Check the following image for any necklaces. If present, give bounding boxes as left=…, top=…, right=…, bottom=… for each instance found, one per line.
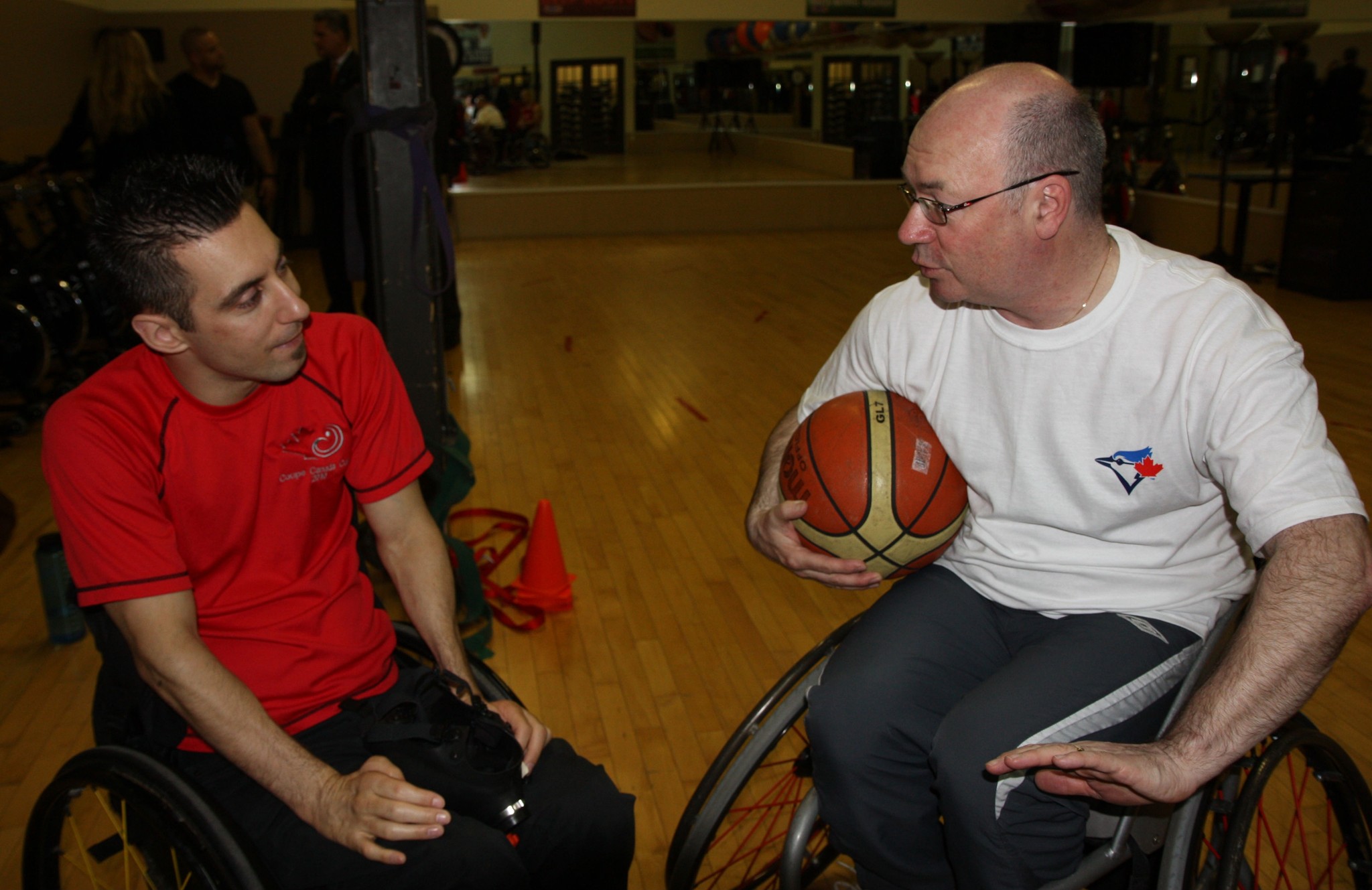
left=1058, top=233, right=1112, bottom=327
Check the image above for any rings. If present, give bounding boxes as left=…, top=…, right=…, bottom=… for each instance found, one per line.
left=1074, top=744, right=1084, bottom=750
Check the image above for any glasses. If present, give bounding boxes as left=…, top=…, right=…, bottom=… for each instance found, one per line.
left=897, top=169, right=1081, bottom=226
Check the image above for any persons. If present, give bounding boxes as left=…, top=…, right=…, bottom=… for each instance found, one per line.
left=40, top=161, right=636, bottom=889
left=741, top=63, right=1372, bottom=890
left=35, top=9, right=1318, bottom=364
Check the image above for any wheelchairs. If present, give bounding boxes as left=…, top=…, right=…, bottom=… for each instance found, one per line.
left=20, top=473, right=553, bottom=890
left=0, top=168, right=135, bottom=442
left=662, top=550, right=1372, bottom=890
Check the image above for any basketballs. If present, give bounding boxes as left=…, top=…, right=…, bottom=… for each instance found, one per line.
left=779, top=390, right=967, bottom=580
